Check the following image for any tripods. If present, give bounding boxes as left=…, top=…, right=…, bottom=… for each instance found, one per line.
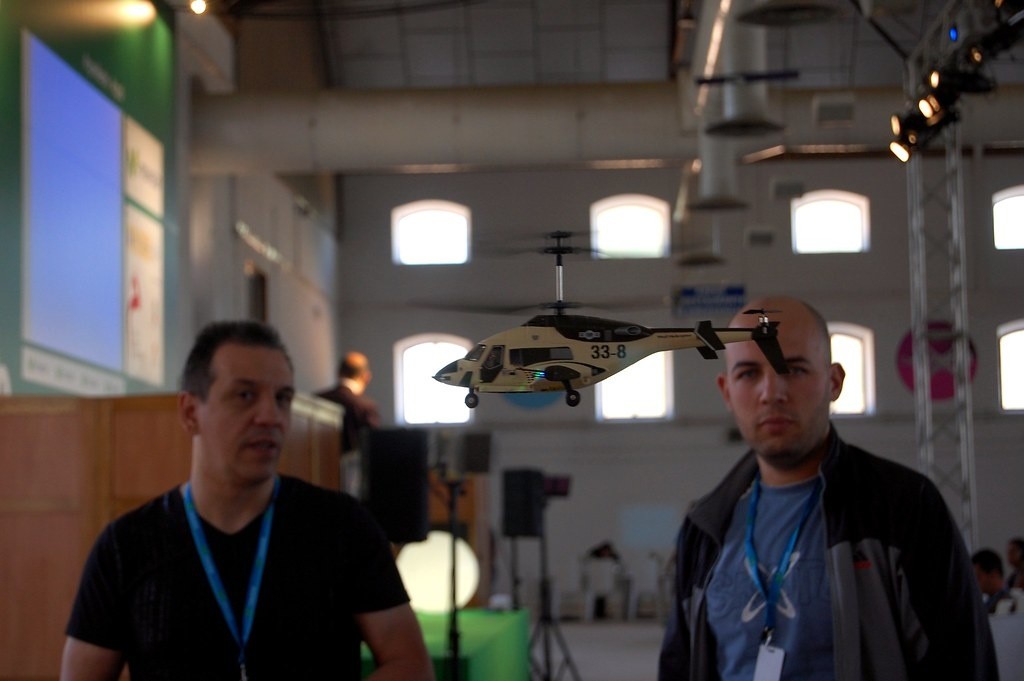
left=528, top=518, right=580, bottom=681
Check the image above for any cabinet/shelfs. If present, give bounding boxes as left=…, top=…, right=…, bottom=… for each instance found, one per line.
left=0, top=386, right=344, bottom=681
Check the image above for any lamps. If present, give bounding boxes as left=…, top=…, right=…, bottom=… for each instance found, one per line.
left=890, top=22, right=1017, bottom=162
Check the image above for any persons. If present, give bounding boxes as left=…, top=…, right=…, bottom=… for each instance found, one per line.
left=972, top=549, right=1015, bottom=612
left=1007, top=539, right=1024, bottom=589
left=658, top=295, right=1002, bottom=681
left=318, top=351, right=381, bottom=449
left=58, top=323, right=435, bottom=681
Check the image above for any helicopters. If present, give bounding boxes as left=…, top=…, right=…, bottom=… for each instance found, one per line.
left=430, top=225, right=794, bottom=407
